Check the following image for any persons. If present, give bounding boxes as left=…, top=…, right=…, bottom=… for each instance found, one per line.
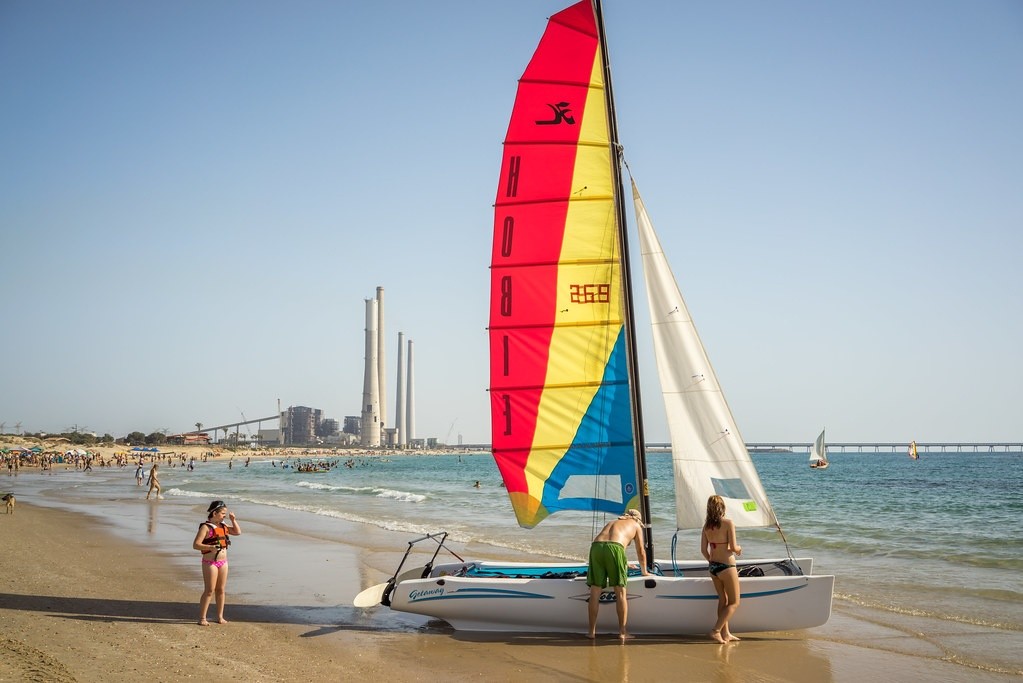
left=74, top=450, right=79, bottom=458
left=245, top=457, right=250, bottom=467
left=229, top=458, right=233, bottom=469
left=459, top=456, right=461, bottom=462
left=146, top=464, right=160, bottom=499
left=473, top=481, right=480, bottom=487
left=251, top=448, right=389, bottom=472
left=501, top=483, right=505, bottom=487
left=193, top=500, right=241, bottom=626
left=135, top=465, right=145, bottom=485
left=585, top=509, right=652, bottom=639
left=817, top=460, right=826, bottom=466
left=0, top=451, right=220, bottom=473
left=701, top=495, right=742, bottom=643
left=2, top=493, right=15, bottom=514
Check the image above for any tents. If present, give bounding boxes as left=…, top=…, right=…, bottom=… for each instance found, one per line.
left=2, top=445, right=42, bottom=453
left=66, top=450, right=75, bottom=455
left=78, top=449, right=87, bottom=455
left=132, top=446, right=159, bottom=451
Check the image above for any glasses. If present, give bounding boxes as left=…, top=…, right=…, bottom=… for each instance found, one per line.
left=211, top=501, right=224, bottom=513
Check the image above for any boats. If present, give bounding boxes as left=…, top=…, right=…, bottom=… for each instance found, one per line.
left=293, top=470, right=328, bottom=473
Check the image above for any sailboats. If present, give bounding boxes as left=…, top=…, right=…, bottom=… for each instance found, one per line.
left=808, top=426, right=829, bottom=469
left=354, top=0, right=832, bottom=633
left=909, top=441, right=919, bottom=460
